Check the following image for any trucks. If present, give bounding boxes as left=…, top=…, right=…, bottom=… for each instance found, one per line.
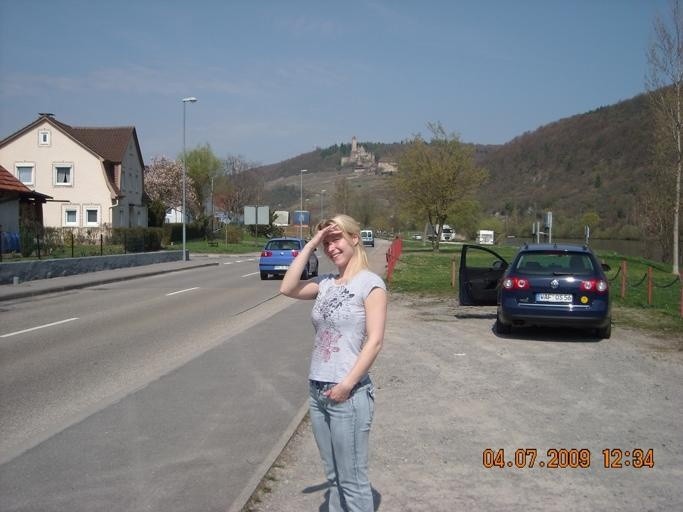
left=426, top=223, right=453, bottom=241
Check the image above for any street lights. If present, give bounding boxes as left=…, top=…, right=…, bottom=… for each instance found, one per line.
left=182, top=96, right=198, bottom=262
left=301, top=169, right=306, bottom=237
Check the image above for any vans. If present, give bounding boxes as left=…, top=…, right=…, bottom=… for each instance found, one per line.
left=360, top=229, right=375, bottom=247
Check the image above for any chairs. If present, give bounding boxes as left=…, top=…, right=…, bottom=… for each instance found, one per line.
left=270, top=244, right=279, bottom=249
left=526, top=255, right=584, bottom=272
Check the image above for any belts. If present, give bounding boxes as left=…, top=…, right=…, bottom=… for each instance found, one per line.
left=308, top=380, right=361, bottom=390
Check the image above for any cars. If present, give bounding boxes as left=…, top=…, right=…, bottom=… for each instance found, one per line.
left=258, top=237, right=319, bottom=280
left=458, top=243, right=611, bottom=340
left=412, top=234, right=422, bottom=240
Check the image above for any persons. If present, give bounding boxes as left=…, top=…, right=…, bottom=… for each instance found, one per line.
left=280, top=213, right=387, bottom=512
